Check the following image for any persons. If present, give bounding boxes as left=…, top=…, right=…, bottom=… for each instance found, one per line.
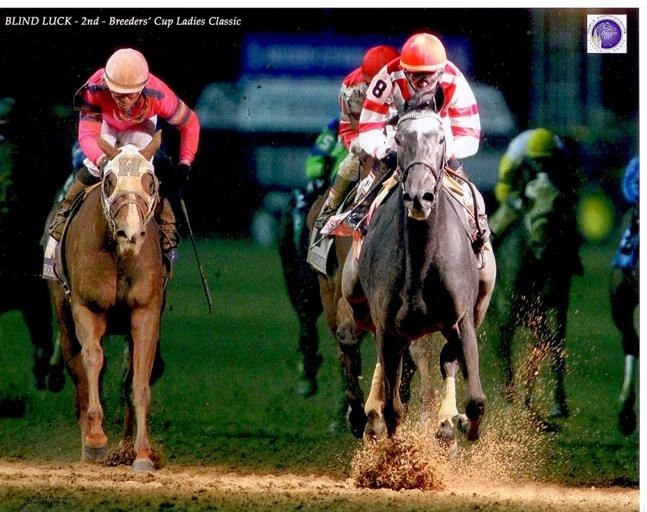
left=611, top=151, right=639, bottom=274
left=348, top=31, right=484, bottom=246
left=41, top=47, right=202, bottom=260
left=307, top=44, right=401, bottom=232
left=485, top=126, right=585, bottom=275
left=296, top=115, right=348, bottom=214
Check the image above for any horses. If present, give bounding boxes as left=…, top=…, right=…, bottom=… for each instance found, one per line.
left=307, top=90, right=433, bottom=438
left=610, top=268, right=640, bottom=436
left=271, top=178, right=363, bottom=399
left=485, top=153, right=577, bottom=433
left=357, top=81, right=497, bottom=462
left=47, top=129, right=164, bottom=473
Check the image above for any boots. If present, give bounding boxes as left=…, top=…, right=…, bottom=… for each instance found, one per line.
left=315, top=174, right=357, bottom=230
left=350, top=159, right=388, bottom=224
left=48, top=175, right=87, bottom=242
left=451, top=162, right=490, bottom=241
left=154, top=181, right=178, bottom=250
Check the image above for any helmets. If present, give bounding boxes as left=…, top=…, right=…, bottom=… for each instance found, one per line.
left=527, top=129, right=553, bottom=157
left=399, top=32, right=447, bottom=73
left=103, top=48, right=150, bottom=94
left=362, top=46, right=401, bottom=77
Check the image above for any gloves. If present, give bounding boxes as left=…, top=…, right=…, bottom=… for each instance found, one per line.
left=160, top=165, right=190, bottom=196
